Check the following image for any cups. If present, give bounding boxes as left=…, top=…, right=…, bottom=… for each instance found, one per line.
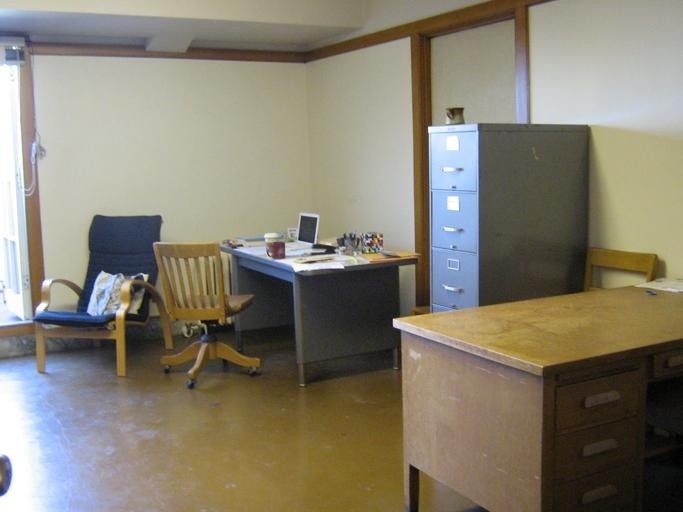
left=266, top=242, right=285, bottom=259
left=445, top=107, right=465, bottom=125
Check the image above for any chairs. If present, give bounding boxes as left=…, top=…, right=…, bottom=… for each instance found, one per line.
left=32, top=214, right=173, bottom=378
left=152, top=242, right=262, bottom=390
left=582, top=246, right=659, bottom=293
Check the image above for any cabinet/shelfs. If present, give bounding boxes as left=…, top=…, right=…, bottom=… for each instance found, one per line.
left=427, top=123, right=588, bottom=314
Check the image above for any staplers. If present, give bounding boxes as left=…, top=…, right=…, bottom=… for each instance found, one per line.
left=311, top=244, right=336, bottom=255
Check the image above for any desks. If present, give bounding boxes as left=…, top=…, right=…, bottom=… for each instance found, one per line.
left=219, top=239, right=417, bottom=388
left=391, top=282, right=681, bottom=512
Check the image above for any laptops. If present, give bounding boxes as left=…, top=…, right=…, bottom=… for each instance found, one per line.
left=285, top=213, right=320, bottom=251
